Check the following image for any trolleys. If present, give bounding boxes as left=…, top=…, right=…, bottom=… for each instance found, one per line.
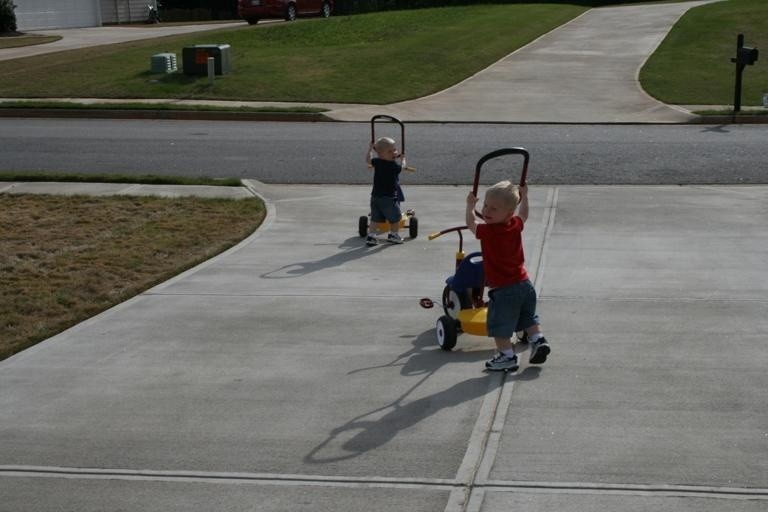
left=357, top=113, right=421, bottom=240
left=418, top=144, right=531, bottom=353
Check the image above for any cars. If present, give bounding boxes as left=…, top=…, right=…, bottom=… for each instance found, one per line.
left=236, top=0, right=337, bottom=26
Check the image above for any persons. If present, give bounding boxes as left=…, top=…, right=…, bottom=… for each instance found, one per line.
left=465, top=179, right=549, bottom=370
left=366, top=136, right=404, bottom=246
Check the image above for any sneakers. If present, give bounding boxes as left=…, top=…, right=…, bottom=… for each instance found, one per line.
left=385, top=234, right=403, bottom=244
left=485, top=352, right=518, bottom=371
left=528, top=337, right=550, bottom=364
left=365, top=236, right=378, bottom=245
